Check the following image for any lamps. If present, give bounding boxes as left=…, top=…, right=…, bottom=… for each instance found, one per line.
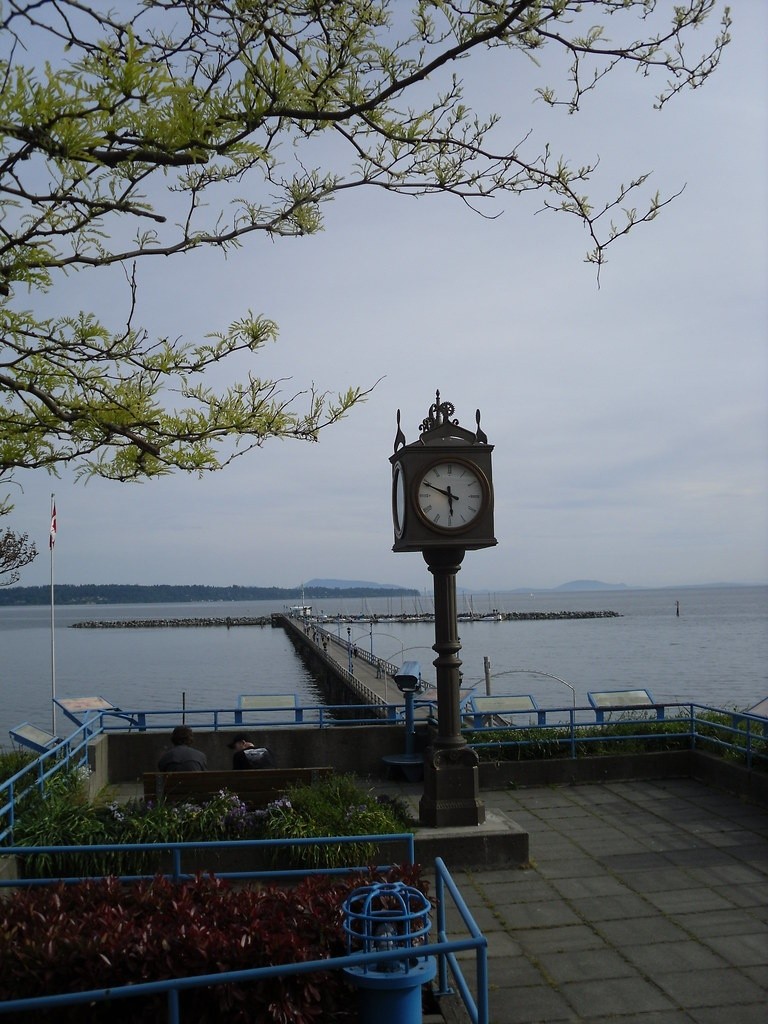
left=338, top=881, right=437, bottom=990
left=394, top=660, right=423, bottom=693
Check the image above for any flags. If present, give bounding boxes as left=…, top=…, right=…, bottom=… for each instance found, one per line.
left=50, top=499, right=57, bottom=551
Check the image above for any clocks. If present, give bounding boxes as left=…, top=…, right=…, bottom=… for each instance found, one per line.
left=411, top=456, right=492, bottom=537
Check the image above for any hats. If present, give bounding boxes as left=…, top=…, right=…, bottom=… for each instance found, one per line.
left=226, top=733, right=255, bottom=750
left=171, top=726, right=193, bottom=742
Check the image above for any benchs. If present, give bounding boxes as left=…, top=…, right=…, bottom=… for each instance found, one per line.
left=141, top=766, right=333, bottom=817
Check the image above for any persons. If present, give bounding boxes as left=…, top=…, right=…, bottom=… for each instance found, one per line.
left=304, top=625, right=310, bottom=636
left=158, top=725, right=209, bottom=772
left=376, top=659, right=382, bottom=679
left=226, top=732, right=274, bottom=770
left=350, top=644, right=357, bottom=658
left=312, top=628, right=319, bottom=642
left=289, top=613, right=294, bottom=619
left=322, top=635, right=330, bottom=648
